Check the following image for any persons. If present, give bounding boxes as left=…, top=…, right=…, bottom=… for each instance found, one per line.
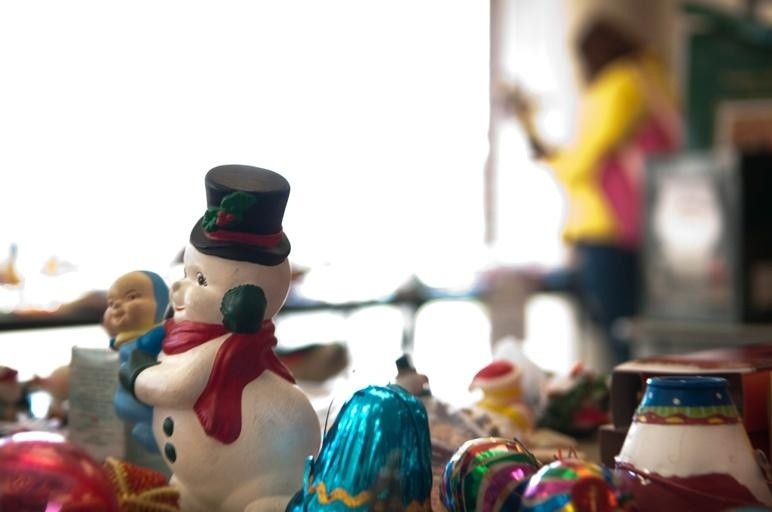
left=102, top=271, right=174, bottom=478
left=500, top=17, right=686, bottom=363
